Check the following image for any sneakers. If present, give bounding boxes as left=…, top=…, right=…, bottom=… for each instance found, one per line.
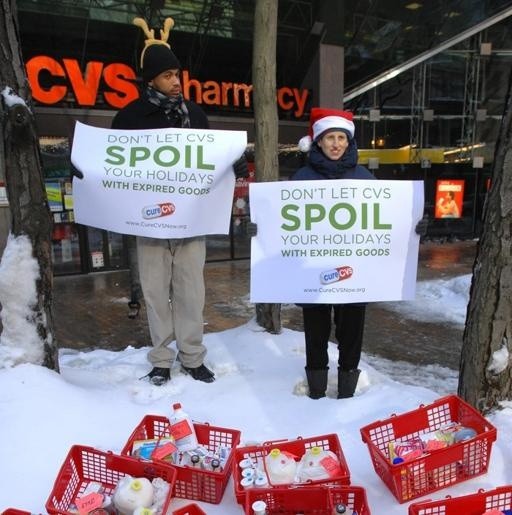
left=179, top=363, right=216, bottom=383
left=148, top=368, right=168, bottom=385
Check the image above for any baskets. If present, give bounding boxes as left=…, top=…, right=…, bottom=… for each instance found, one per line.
left=46, top=444, right=177, bottom=515
left=407, top=485, right=512, bottom=515
left=360, top=395, right=497, bottom=504
left=120, top=415, right=242, bottom=504
left=232, top=433, right=373, bottom=515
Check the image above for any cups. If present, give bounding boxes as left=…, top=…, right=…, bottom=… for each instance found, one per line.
left=455, top=428, right=480, bottom=475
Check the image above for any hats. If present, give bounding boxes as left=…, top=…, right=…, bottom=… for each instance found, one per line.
left=298, top=107, right=355, bottom=152
left=143, top=45, right=181, bottom=83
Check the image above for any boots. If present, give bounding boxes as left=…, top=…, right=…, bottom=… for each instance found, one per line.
left=304, top=366, right=328, bottom=399
left=338, top=367, right=361, bottom=398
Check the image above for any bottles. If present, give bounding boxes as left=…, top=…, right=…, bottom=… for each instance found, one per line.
left=334, top=504, right=353, bottom=515
left=239, top=446, right=345, bottom=507
left=202, top=460, right=221, bottom=503
left=187, top=455, right=202, bottom=500
left=252, top=500, right=267, bottom=515
left=393, top=457, right=413, bottom=501
left=169, top=403, right=198, bottom=453
left=89, top=477, right=166, bottom=515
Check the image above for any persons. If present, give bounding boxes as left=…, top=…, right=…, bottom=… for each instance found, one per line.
left=69, top=44, right=250, bottom=385
left=246, top=107, right=429, bottom=399
left=436, top=191, right=459, bottom=218
